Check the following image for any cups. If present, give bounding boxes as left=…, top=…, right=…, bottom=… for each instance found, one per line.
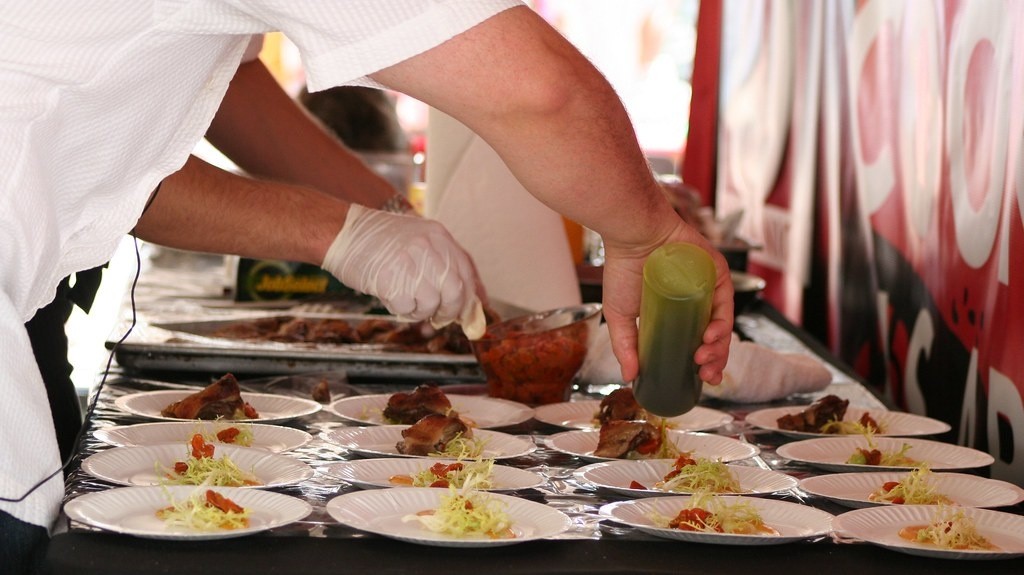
left=631, top=241, right=717, bottom=417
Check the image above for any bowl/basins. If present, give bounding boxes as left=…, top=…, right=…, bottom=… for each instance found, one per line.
left=472, top=302, right=603, bottom=403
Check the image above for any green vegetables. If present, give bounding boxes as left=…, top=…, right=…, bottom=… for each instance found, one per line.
left=148, top=413, right=995, bottom=549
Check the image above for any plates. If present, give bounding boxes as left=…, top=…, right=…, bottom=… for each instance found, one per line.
left=93, top=422, right=313, bottom=447
left=775, top=438, right=995, bottom=468
left=81, top=443, right=314, bottom=488
left=747, top=407, right=950, bottom=436
left=317, top=424, right=538, bottom=459
left=598, top=495, right=837, bottom=545
left=535, top=399, right=734, bottom=431
left=72, top=488, right=313, bottom=540
left=546, top=430, right=761, bottom=463
left=834, top=506, right=1024, bottom=560
left=115, top=389, right=323, bottom=423
left=576, top=459, right=799, bottom=496
left=330, top=393, right=535, bottom=428
left=328, top=459, right=544, bottom=490
left=326, top=487, right=572, bottom=548
left=799, top=471, right=1024, bottom=506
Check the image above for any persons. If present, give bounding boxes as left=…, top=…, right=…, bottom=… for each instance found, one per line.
left=22, top=34, right=487, bottom=481
left=0, top=0, right=734, bottom=575
